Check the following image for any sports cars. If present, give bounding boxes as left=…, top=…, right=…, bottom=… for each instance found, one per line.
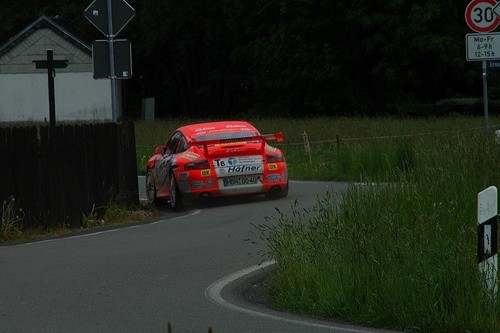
left=146, top=120, right=288, bottom=211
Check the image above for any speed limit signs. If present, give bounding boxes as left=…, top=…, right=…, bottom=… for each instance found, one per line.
left=464, top=0, right=500, bottom=33
